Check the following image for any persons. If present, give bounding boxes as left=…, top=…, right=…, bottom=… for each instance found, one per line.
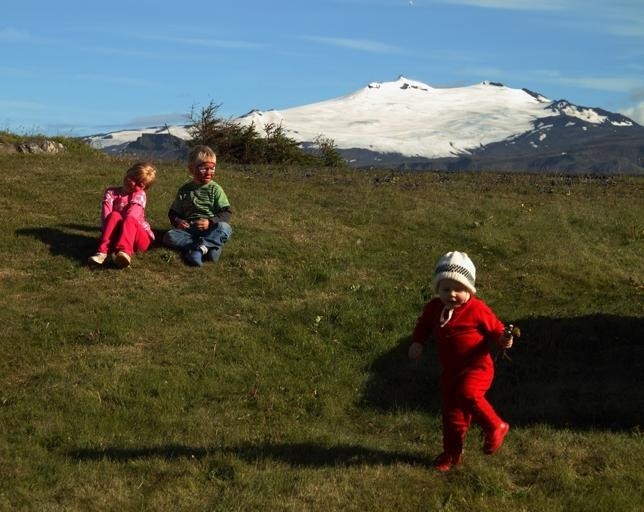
left=408, top=251, right=522, bottom=472
left=88, top=162, right=157, bottom=269
left=162, top=145, right=233, bottom=267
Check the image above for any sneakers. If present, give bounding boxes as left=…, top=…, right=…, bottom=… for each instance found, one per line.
left=185, top=245, right=203, bottom=266
left=435, top=452, right=462, bottom=472
left=115, top=251, right=131, bottom=270
left=208, top=248, right=221, bottom=262
left=483, top=422, right=509, bottom=454
left=89, top=252, right=110, bottom=266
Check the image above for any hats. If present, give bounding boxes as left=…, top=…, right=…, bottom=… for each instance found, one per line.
left=432, top=251, right=477, bottom=294
